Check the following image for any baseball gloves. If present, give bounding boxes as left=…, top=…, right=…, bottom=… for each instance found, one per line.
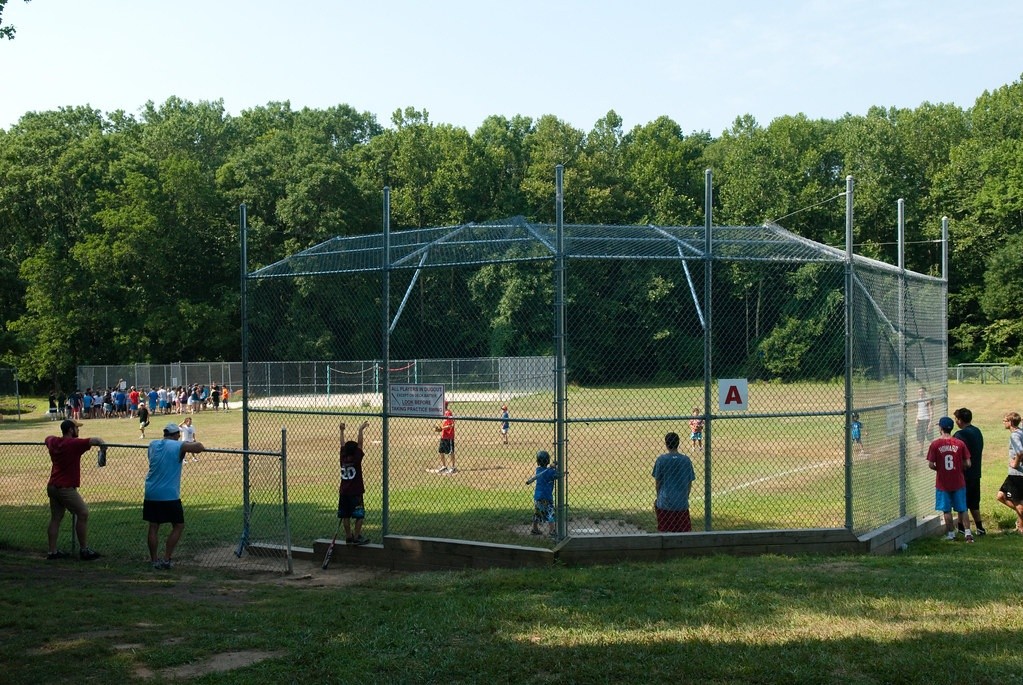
left=431, top=425, right=442, bottom=432
left=694, top=425, right=703, bottom=432
left=144, top=421, right=150, bottom=427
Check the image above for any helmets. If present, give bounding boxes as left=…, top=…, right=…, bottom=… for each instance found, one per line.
left=501, top=405, right=507, bottom=412
left=536, top=451, right=550, bottom=466
left=851, top=412, right=859, bottom=420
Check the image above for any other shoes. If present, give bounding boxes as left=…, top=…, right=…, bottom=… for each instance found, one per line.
left=549, top=529, right=556, bottom=535
left=449, top=467, right=456, bottom=473
left=353, top=534, right=371, bottom=544
left=346, top=533, right=354, bottom=544
left=163, top=560, right=170, bottom=569
left=439, top=466, right=447, bottom=472
left=184, top=458, right=188, bottom=463
left=193, top=457, right=198, bottom=461
left=917, top=453, right=924, bottom=457
left=140, top=435, right=145, bottom=439
left=531, top=528, right=542, bottom=534
left=151, top=558, right=161, bottom=568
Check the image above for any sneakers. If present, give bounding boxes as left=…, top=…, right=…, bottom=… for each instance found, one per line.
left=79, top=548, right=102, bottom=559
left=947, top=526, right=987, bottom=543
left=47, top=549, right=68, bottom=558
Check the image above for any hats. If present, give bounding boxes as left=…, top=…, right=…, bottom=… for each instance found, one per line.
left=61, top=419, right=83, bottom=430
left=140, top=402, right=144, bottom=406
left=163, top=423, right=183, bottom=434
left=444, top=400, right=449, bottom=406
left=936, top=417, right=954, bottom=429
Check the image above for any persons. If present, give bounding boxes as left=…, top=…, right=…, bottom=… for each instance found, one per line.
left=434, top=400, right=457, bottom=473
left=689, top=408, right=704, bottom=452
left=142, top=423, right=204, bottom=571
left=44, top=419, right=107, bottom=561
left=852, top=412, right=868, bottom=454
left=925, top=416, right=974, bottom=540
left=500, top=405, right=509, bottom=444
left=652, top=432, right=696, bottom=533
left=996, top=412, right=1023, bottom=532
left=337, top=420, right=371, bottom=544
left=952, top=407, right=986, bottom=536
left=49, top=378, right=230, bottom=421
left=177, top=417, right=198, bottom=464
left=913, top=386, right=936, bottom=457
left=136, top=402, right=150, bottom=439
left=530, top=451, right=557, bottom=537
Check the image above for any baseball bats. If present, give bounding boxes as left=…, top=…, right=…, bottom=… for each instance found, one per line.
left=526, top=461, right=558, bottom=485
